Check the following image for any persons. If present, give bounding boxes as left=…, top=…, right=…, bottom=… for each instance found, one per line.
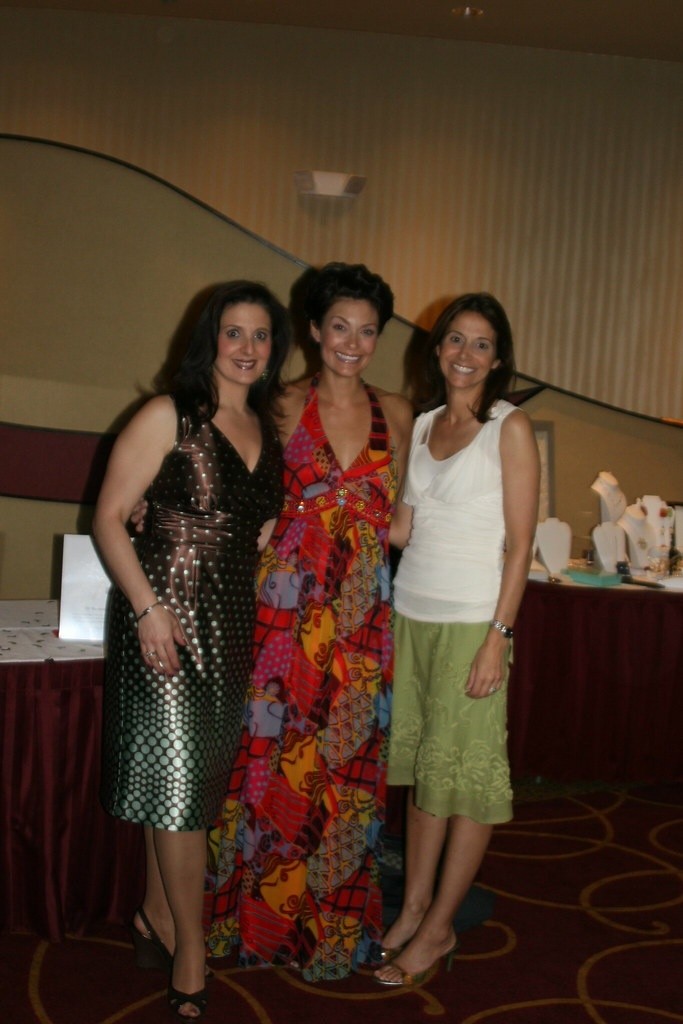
left=93, top=261, right=542, bottom=1019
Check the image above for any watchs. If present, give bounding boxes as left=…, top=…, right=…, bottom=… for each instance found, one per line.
left=491, top=619, right=514, bottom=639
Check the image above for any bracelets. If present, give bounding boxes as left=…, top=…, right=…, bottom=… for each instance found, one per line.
left=136, top=602, right=161, bottom=622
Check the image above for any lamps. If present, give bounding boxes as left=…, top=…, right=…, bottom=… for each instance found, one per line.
left=294, top=170, right=367, bottom=199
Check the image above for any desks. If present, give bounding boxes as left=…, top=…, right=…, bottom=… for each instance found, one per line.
left=1, top=599, right=149, bottom=934
left=506, top=565, right=682, bottom=800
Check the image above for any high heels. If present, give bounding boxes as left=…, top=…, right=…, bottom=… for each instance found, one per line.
left=130, top=907, right=215, bottom=982
left=350, top=939, right=461, bottom=985
left=167, top=977, right=209, bottom=1023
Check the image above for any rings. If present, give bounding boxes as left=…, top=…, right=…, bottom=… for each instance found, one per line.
left=489, top=688, right=496, bottom=693
left=146, top=652, right=156, bottom=658
